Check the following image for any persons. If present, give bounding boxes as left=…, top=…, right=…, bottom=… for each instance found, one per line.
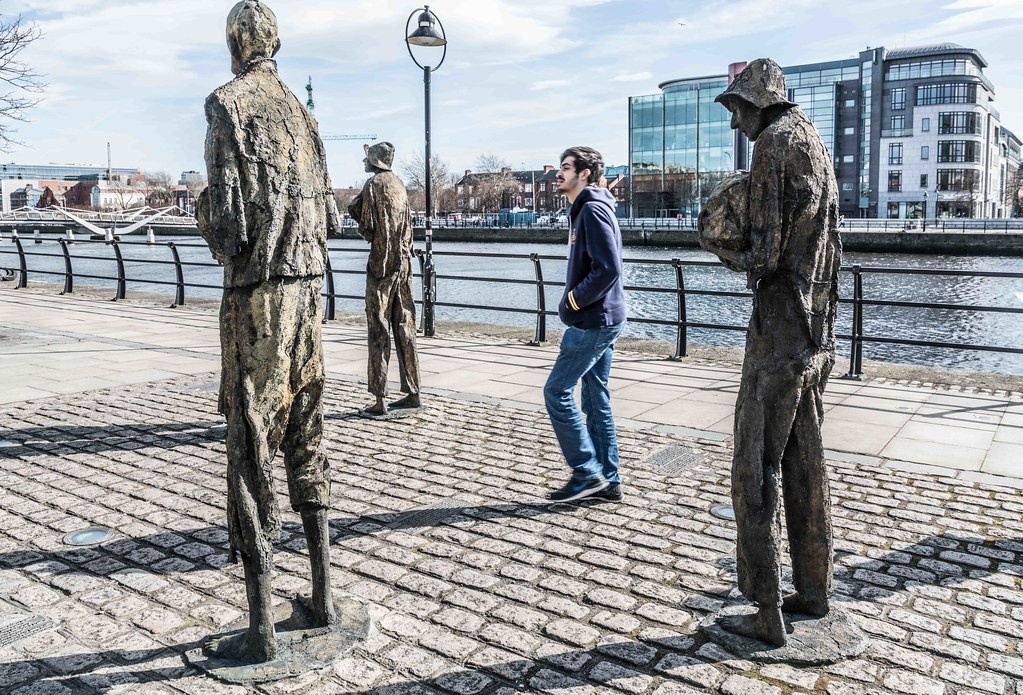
left=698, top=58, right=841, bottom=646
left=193, top=1, right=340, bottom=663
left=346, top=142, right=421, bottom=416
left=543, top=147, right=628, bottom=505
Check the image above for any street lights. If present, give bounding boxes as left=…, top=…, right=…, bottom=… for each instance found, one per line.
left=522, top=162, right=535, bottom=212
left=404, top=3, right=447, bottom=340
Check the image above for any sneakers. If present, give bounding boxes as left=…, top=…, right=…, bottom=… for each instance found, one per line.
left=545, top=478, right=610, bottom=502
left=577, top=483, right=624, bottom=502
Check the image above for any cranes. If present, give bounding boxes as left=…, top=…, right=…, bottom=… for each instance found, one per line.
left=319, top=134, right=378, bottom=141
